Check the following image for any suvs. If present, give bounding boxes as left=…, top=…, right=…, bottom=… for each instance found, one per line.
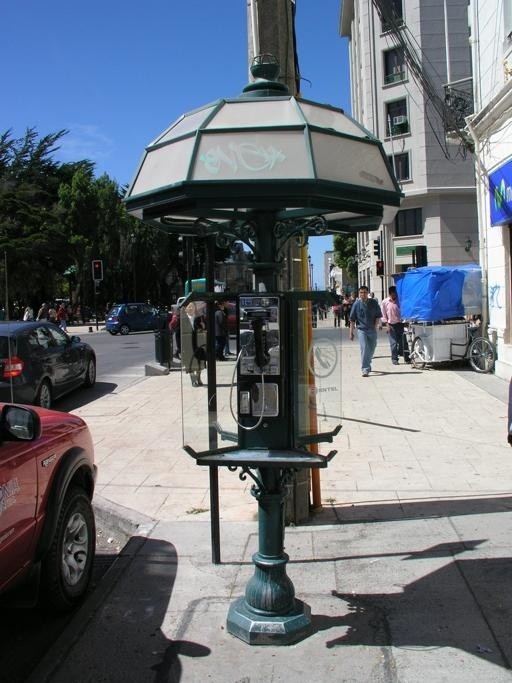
left=0, top=403, right=97, bottom=642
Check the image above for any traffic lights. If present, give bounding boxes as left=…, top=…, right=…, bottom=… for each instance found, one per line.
left=91, top=260, right=104, bottom=282
left=374, top=237, right=380, bottom=256
left=376, top=261, right=385, bottom=274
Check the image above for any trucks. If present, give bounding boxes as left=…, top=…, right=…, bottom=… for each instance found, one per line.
left=240, top=299, right=273, bottom=326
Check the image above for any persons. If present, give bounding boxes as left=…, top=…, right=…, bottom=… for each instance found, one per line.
left=331, top=290, right=382, bottom=328
left=105, top=302, right=117, bottom=315
left=13, top=302, right=68, bottom=333
left=214, top=298, right=234, bottom=360
left=153, top=301, right=207, bottom=387
left=381, top=286, right=412, bottom=365
left=349, top=286, right=383, bottom=376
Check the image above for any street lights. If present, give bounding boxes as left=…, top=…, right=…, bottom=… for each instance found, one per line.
left=308, top=253, right=319, bottom=292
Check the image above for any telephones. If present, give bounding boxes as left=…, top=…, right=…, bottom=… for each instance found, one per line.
left=251, top=318, right=270, bottom=367
left=192, top=315, right=206, bottom=360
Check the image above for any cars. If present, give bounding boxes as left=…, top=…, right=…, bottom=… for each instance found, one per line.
left=103, top=297, right=238, bottom=336
left=0, top=319, right=98, bottom=409
left=1, top=297, right=72, bottom=312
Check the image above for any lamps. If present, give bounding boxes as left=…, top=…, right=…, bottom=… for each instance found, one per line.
left=464, top=239, right=472, bottom=252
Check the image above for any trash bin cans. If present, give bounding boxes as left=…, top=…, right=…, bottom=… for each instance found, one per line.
left=154, top=328, right=173, bottom=371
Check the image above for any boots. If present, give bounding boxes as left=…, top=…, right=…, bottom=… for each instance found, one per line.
left=190, top=372, right=203, bottom=387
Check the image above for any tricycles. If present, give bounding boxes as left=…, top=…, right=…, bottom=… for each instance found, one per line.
left=406, top=315, right=497, bottom=376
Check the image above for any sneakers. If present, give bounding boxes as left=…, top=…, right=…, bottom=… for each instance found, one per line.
left=392, top=359, right=398, bottom=365
left=173, top=353, right=181, bottom=360
left=361, top=366, right=371, bottom=377
left=216, top=352, right=234, bottom=361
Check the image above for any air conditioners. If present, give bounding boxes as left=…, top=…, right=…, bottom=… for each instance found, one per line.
left=393, top=115, right=407, bottom=126
